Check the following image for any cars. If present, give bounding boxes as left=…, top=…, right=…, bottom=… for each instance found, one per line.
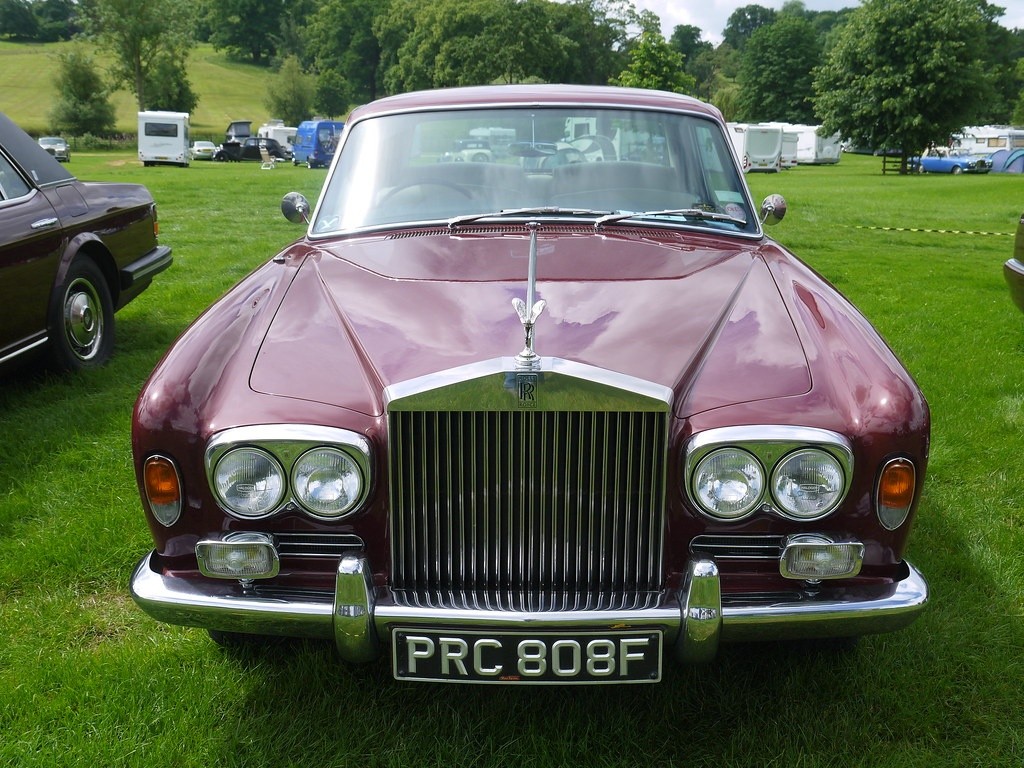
left=841, top=137, right=902, bottom=157
left=128, top=82, right=933, bottom=686
left=37, top=137, right=72, bottom=164
left=0, top=112, right=174, bottom=378
left=445, top=138, right=496, bottom=164
left=189, top=140, right=216, bottom=161
left=908, top=146, right=994, bottom=175
left=240, top=138, right=285, bottom=163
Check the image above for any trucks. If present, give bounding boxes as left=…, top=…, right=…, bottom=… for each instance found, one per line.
left=258, top=127, right=298, bottom=154
left=287, top=120, right=345, bottom=169
left=471, top=127, right=515, bottom=157
left=694, top=121, right=842, bottom=174
left=137, top=110, right=194, bottom=168
left=563, top=117, right=650, bottom=161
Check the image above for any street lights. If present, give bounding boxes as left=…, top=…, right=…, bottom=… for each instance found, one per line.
left=531, top=113, right=535, bottom=148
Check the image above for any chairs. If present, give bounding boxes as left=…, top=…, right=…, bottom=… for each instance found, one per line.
left=548, top=162, right=680, bottom=216
left=398, top=162, right=529, bottom=215
